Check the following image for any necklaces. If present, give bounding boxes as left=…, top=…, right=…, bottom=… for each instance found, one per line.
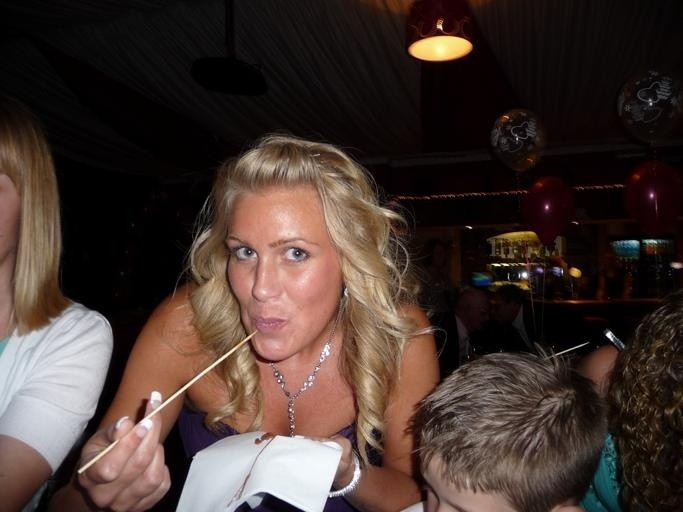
left=262, top=316, right=343, bottom=438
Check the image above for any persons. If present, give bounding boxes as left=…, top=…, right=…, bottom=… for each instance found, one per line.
left=0, top=103, right=115, bottom=511
left=418, top=238, right=449, bottom=281
left=42, top=131, right=440, bottom=512
left=573, top=300, right=681, bottom=511
left=476, top=286, right=536, bottom=353
left=402, top=350, right=610, bottom=512
left=598, top=268, right=632, bottom=300
left=436, top=287, right=487, bottom=367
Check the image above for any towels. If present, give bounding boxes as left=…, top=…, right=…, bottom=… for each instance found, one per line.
left=175, top=431, right=343, bottom=512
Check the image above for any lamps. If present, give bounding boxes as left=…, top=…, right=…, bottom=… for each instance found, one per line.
left=406, top=0, right=474, bottom=63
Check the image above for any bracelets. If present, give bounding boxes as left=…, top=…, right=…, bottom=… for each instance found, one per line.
left=327, top=452, right=363, bottom=500
